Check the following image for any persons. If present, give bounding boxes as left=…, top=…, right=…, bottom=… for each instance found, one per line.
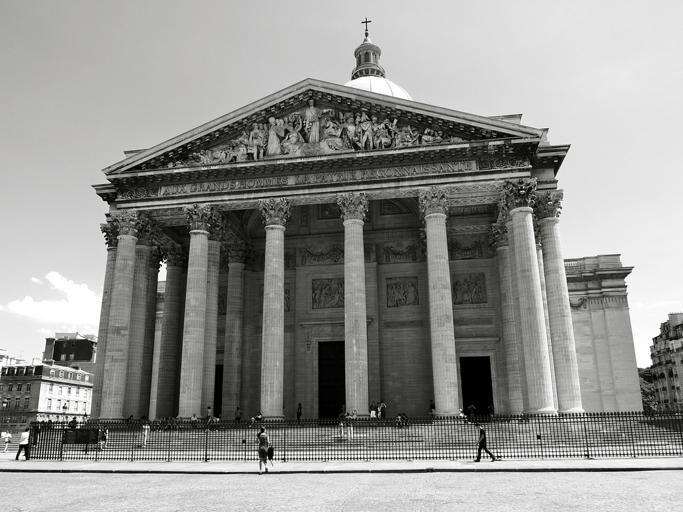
left=282, top=237, right=488, bottom=314
left=467, top=403, right=476, bottom=423
left=296, top=402, right=302, bottom=425
left=473, top=422, right=495, bottom=462
left=256, top=426, right=270, bottom=475
left=166, top=99, right=452, bottom=169
left=344, top=411, right=354, bottom=440
left=0, top=399, right=224, bottom=462
left=367, top=399, right=435, bottom=428
left=233, top=405, right=261, bottom=429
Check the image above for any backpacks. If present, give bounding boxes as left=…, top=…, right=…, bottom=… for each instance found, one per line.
left=267, top=447, right=274, bottom=460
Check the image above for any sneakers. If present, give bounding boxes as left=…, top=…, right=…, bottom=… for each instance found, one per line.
left=474, top=460, right=480, bottom=462
left=265, top=467, right=268, bottom=473
left=492, top=458, right=495, bottom=462
